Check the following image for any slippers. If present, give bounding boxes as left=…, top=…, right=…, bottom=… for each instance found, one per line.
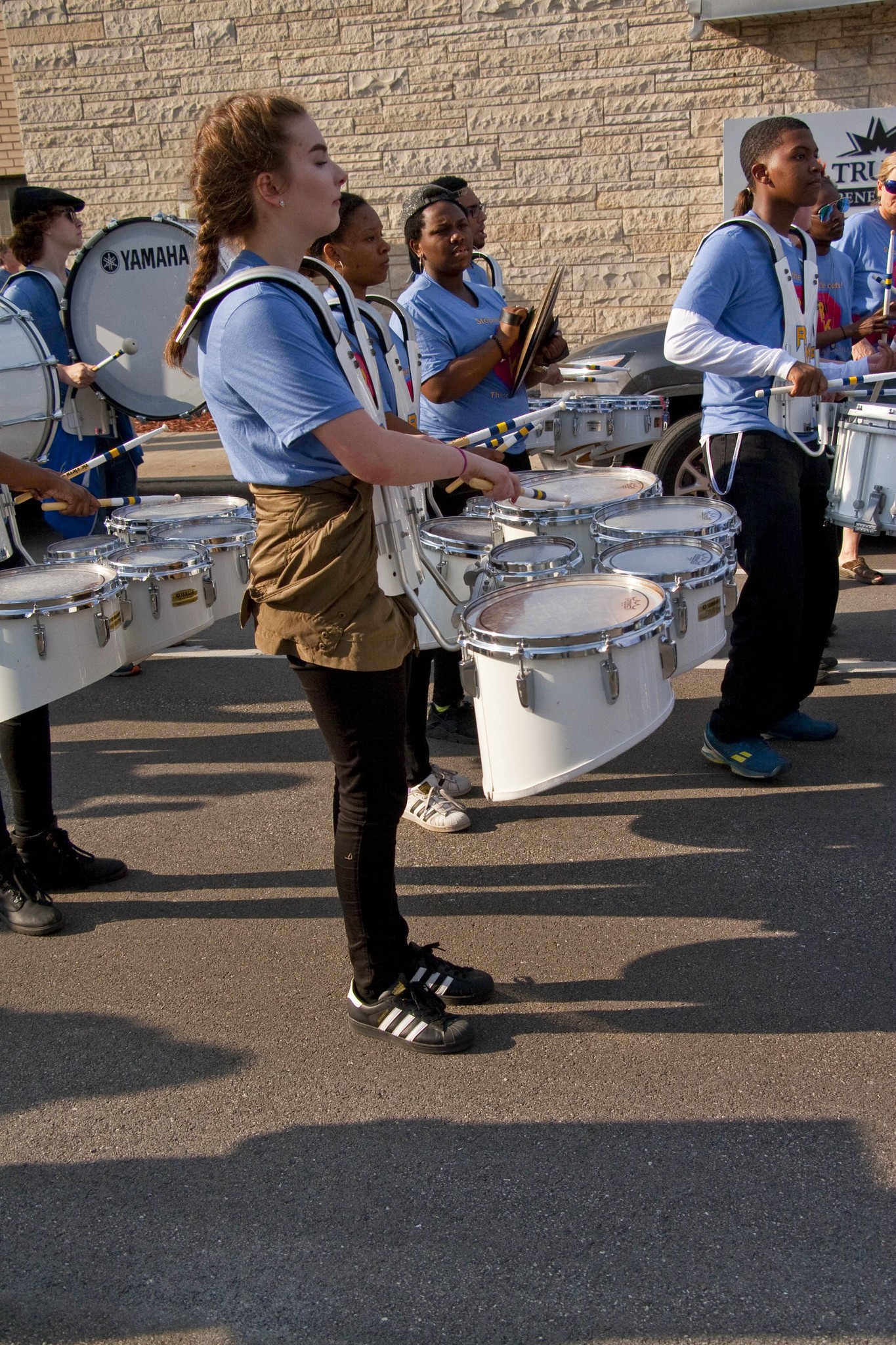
left=839, top=555, right=885, bottom=584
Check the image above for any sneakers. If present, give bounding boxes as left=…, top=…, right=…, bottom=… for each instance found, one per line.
left=347, top=976, right=474, bottom=1054
left=423, top=760, right=472, bottom=797
left=107, top=662, right=142, bottom=676
left=400, top=775, right=472, bottom=832
left=762, top=706, right=840, bottom=745
left=702, top=722, right=787, bottom=780
left=384, top=942, right=496, bottom=1005
left=426, top=696, right=479, bottom=744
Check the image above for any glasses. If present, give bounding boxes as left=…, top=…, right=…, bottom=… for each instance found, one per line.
left=56, top=207, right=77, bottom=223
left=810, top=198, right=852, bottom=223
left=466, top=202, right=488, bottom=219
left=881, top=179, right=895, bottom=194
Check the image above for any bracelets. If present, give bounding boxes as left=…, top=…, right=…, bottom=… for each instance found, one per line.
left=453, top=445, right=468, bottom=477
left=840, top=325, right=847, bottom=340
left=490, top=334, right=506, bottom=362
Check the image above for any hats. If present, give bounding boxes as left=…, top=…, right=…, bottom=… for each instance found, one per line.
left=8, top=185, right=86, bottom=229
left=399, top=184, right=461, bottom=275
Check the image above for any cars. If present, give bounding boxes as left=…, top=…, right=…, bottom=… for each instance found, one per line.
left=528, top=319, right=721, bottom=501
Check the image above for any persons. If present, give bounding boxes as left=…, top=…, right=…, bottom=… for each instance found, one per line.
left=163, top=92, right=511, bottom=1054
left=0, top=114, right=896, bottom=946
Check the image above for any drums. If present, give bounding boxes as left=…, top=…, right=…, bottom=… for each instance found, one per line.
left=527, top=399, right=555, bottom=451
left=64, top=213, right=234, bottom=425
left=0, top=558, right=115, bottom=720
left=461, top=494, right=494, bottom=517
left=594, top=534, right=736, bottom=678
left=576, top=395, right=665, bottom=460
left=0, top=293, right=67, bottom=480
left=409, top=514, right=495, bottom=652
left=44, top=532, right=126, bottom=565
left=454, top=568, right=676, bottom=806
left=104, top=537, right=216, bottom=665
left=823, top=400, right=896, bottom=538
left=106, top=491, right=254, bottom=547
left=590, top=492, right=740, bottom=559
left=144, top=515, right=257, bottom=621
left=511, top=468, right=563, bottom=479
left=486, top=534, right=583, bottom=588
left=491, top=466, right=664, bottom=573
left=551, top=396, right=614, bottom=462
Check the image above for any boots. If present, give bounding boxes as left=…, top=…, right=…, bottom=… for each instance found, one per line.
left=8, top=814, right=128, bottom=888
left=0, top=844, right=64, bottom=936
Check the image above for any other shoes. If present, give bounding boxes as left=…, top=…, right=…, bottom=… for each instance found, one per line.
left=818, top=656, right=838, bottom=671
left=816, top=670, right=830, bottom=684
